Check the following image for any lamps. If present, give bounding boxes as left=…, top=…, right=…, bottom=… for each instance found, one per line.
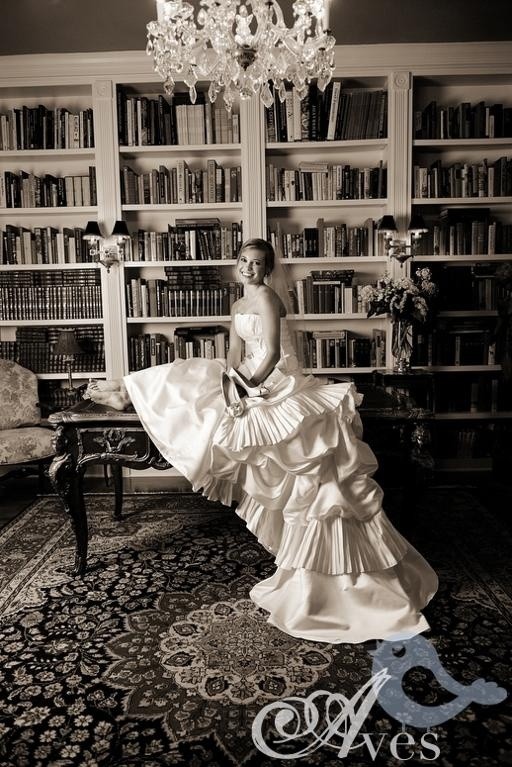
left=145, top=0, right=337, bottom=119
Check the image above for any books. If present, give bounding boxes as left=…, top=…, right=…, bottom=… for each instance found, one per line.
left=0, top=77, right=511, bottom=462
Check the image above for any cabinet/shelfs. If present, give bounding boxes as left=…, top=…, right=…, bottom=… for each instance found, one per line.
left=0, top=40, right=512, bottom=477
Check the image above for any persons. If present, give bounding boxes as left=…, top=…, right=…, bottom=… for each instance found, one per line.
left=85, top=239, right=439, bottom=644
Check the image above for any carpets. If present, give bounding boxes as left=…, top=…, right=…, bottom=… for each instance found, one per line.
left=0, top=490, right=511, bottom=767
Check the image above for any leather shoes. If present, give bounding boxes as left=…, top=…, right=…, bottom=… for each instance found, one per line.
left=228, top=368, right=270, bottom=397
left=221, top=371, right=244, bottom=417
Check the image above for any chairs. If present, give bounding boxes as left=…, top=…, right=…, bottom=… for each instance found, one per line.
left=0, top=359, right=56, bottom=502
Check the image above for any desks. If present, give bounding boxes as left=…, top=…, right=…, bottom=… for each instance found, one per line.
left=51, top=382, right=434, bottom=582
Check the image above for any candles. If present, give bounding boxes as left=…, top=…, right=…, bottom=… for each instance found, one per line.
left=317, top=0, right=333, bottom=42
